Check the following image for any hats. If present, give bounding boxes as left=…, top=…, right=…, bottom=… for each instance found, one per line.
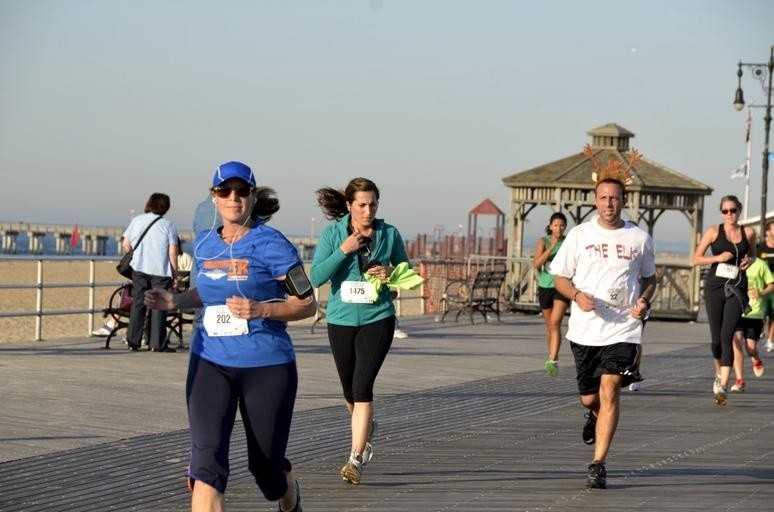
left=213, top=162, right=256, bottom=188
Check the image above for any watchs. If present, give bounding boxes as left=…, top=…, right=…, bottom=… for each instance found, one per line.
left=641, top=297, right=651, bottom=311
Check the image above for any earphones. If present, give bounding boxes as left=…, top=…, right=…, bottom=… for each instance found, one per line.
left=252, top=195, right=258, bottom=204
left=212, top=197, right=216, bottom=204
left=739, top=211, right=741, bottom=214
left=721, top=213, right=723, bottom=216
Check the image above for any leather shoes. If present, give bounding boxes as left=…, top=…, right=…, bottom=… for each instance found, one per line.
left=129, top=344, right=176, bottom=351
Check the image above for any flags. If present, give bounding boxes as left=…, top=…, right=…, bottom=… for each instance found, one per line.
left=69, top=224, right=79, bottom=249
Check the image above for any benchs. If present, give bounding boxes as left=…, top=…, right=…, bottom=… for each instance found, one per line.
left=438, top=269, right=509, bottom=325
left=309, top=291, right=398, bottom=336
left=101, top=267, right=193, bottom=352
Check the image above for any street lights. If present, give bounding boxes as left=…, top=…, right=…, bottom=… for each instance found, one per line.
left=732, top=45, right=774, bottom=242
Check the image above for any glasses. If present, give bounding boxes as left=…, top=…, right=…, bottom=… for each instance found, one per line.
left=722, top=209, right=737, bottom=214
left=211, top=186, right=254, bottom=198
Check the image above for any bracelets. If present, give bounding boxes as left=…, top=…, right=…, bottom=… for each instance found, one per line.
left=574, top=291, right=580, bottom=301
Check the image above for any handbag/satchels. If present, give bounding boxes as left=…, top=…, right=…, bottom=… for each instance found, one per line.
left=116, top=249, right=133, bottom=280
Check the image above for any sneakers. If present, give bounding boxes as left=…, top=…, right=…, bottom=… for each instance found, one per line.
left=341, top=458, right=364, bottom=484
left=713, top=339, right=774, bottom=406
left=582, top=410, right=597, bottom=445
left=362, top=418, right=377, bottom=465
left=586, top=460, right=606, bottom=489
left=629, top=382, right=640, bottom=392
left=92, top=323, right=117, bottom=336
left=393, top=329, right=408, bottom=339
left=545, top=360, right=559, bottom=377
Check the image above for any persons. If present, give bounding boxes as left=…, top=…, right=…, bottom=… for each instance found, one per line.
left=309, top=177, right=412, bottom=485
left=628, top=220, right=660, bottom=391
left=143, top=161, right=318, bottom=512
left=532, top=212, right=567, bottom=378
left=91, top=193, right=194, bottom=353
left=693, top=195, right=774, bottom=406
left=550, top=179, right=656, bottom=489
